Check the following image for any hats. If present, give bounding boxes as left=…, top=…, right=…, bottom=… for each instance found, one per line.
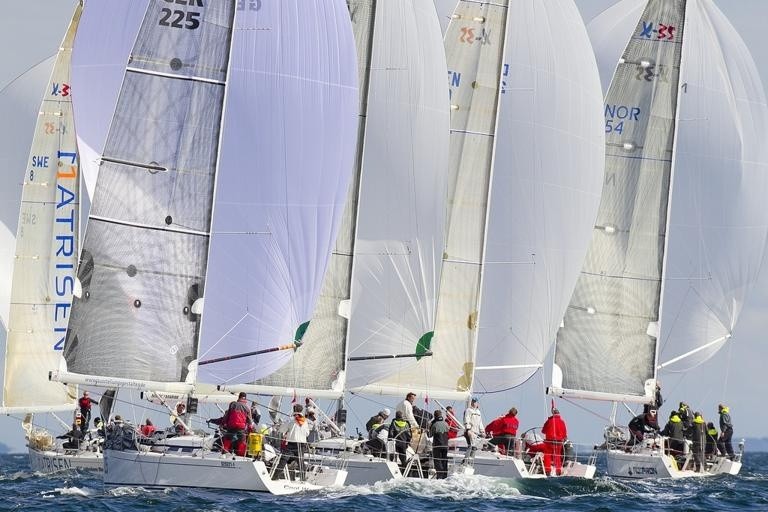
left=472, top=396, right=480, bottom=403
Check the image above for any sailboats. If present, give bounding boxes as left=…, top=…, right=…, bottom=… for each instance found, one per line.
left=347, top=3, right=450, bottom=482
left=2, top=0, right=105, bottom=475
left=554, top=1, right=766, bottom=485
left=48, top=0, right=347, bottom=494
left=487, top=0, right=608, bottom=489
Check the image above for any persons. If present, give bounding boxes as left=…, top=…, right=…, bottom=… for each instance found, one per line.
left=77, top=390, right=98, bottom=437
left=484, top=414, right=509, bottom=456
left=360, top=389, right=460, bottom=484
left=485, top=407, right=519, bottom=456
left=135, top=391, right=337, bottom=479
left=558, top=440, right=577, bottom=469
left=641, top=381, right=664, bottom=426
left=623, top=402, right=737, bottom=474
left=113, top=415, right=124, bottom=430
left=93, top=417, right=104, bottom=431
left=539, top=407, right=567, bottom=476
left=520, top=433, right=545, bottom=464
left=55, top=424, right=83, bottom=452
left=461, top=397, right=486, bottom=467
